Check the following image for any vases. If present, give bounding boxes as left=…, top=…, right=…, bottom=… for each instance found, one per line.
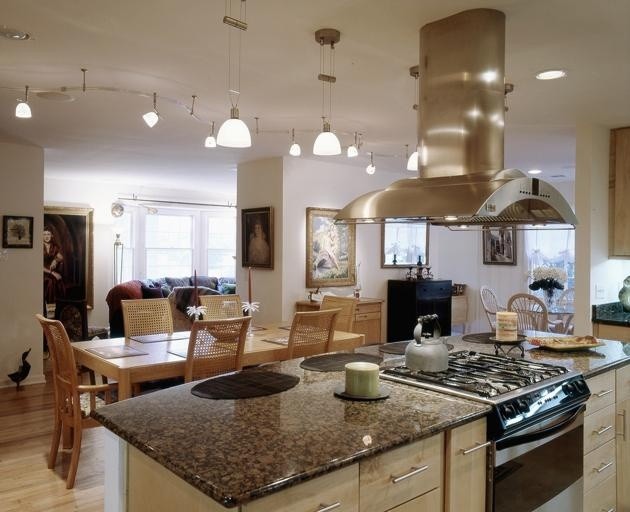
left=247, top=310, right=254, bottom=337
left=547, top=290, right=555, bottom=311
left=194, top=315, right=199, bottom=322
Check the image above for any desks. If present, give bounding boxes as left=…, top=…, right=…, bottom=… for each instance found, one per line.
left=452, top=295, right=470, bottom=335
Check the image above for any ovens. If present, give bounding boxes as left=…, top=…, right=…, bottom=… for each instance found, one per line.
left=486, top=403, right=587, bottom=512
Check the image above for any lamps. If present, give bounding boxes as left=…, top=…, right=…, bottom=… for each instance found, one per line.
left=407, top=151, right=419, bottom=171
left=142, top=93, right=160, bottom=129
left=312, top=29, right=341, bottom=156
left=348, top=132, right=364, bottom=158
left=14, top=101, right=32, bottom=119
left=203, top=120, right=217, bottom=150
left=366, top=151, right=377, bottom=175
left=289, top=129, right=301, bottom=157
left=215, top=0, right=252, bottom=149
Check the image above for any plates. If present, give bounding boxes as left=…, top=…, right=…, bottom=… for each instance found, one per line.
left=334, top=387, right=389, bottom=400
left=541, top=340, right=605, bottom=353
left=489, top=335, right=526, bottom=345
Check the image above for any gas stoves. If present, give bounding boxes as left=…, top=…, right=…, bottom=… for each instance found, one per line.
left=378, top=350, right=591, bottom=438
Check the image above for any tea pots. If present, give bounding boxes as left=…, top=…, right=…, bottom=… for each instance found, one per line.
left=404, top=313, right=450, bottom=373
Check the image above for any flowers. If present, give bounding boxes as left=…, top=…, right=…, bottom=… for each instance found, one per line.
left=241, top=301, right=260, bottom=313
left=524, top=266, right=567, bottom=290
left=186, top=305, right=207, bottom=315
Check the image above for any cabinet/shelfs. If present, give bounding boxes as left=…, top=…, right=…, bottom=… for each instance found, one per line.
left=609, top=126, right=630, bottom=260
left=296, top=297, right=384, bottom=345
left=592, top=302, right=630, bottom=341
left=616, top=363, right=630, bottom=511
left=445, top=417, right=486, bottom=512
left=240, top=464, right=360, bottom=512
left=584, top=370, right=617, bottom=511
left=387, top=279, right=453, bottom=342
left=360, top=431, right=445, bottom=512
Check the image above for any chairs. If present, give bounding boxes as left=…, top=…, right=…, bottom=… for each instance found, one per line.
left=184, top=315, right=252, bottom=382
left=480, top=284, right=507, bottom=332
left=557, top=287, right=575, bottom=335
left=287, top=308, right=344, bottom=359
left=120, top=297, right=174, bottom=336
left=507, top=293, right=549, bottom=332
left=319, top=295, right=356, bottom=331
left=199, top=295, right=244, bottom=319
left=36, top=314, right=141, bottom=490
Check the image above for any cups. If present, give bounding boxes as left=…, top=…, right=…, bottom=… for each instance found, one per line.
left=344, top=361, right=380, bottom=397
left=496, top=312, right=518, bottom=341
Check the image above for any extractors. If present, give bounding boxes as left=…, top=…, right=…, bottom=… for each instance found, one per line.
left=329, top=8, right=579, bottom=231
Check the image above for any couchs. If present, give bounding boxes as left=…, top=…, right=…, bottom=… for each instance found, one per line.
left=107, top=276, right=236, bottom=338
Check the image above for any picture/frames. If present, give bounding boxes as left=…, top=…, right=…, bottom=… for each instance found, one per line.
left=3, top=216, right=33, bottom=249
left=306, top=207, right=358, bottom=288
left=380, top=218, right=431, bottom=269
left=43, top=206, right=95, bottom=312
left=241, top=206, right=275, bottom=271
left=482, top=226, right=518, bottom=266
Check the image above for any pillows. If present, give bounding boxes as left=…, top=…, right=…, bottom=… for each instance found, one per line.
left=166, top=278, right=189, bottom=291
left=142, top=285, right=164, bottom=298
left=162, top=288, right=171, bottom=297
left=191, top=276, right=217, bottom=290
